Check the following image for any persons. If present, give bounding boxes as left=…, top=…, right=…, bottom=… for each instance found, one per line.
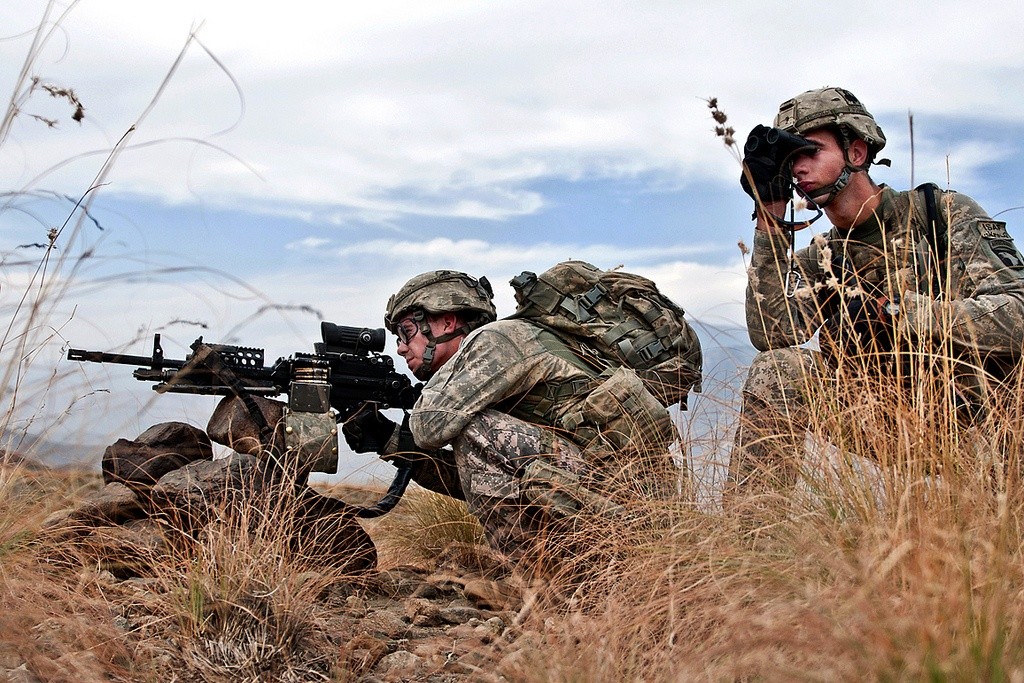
left=341, top=268, right=680, bottom=558
left=723, top=87, right=1024, bottom=546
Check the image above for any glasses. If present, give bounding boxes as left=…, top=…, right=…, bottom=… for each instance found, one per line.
left=396, top=318, right=419, bottom=345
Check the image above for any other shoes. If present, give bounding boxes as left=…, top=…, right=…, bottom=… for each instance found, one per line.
left=462, top=570, right=560, bottom=611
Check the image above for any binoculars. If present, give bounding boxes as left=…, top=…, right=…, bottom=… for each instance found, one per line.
left=745, top=128, right=816, bottom=157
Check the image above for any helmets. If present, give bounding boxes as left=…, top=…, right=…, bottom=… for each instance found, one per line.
left=770, top=87, right=887, bottom=158
left=384, top=270, right=497, bottom=333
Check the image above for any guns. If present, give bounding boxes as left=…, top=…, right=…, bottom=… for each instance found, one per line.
left=67, top=321, right=425, bottom=420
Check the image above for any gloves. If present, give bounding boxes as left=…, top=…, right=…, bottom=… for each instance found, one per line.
left=740, top=124, right=803, bottom=204
left=827, top=298, right=882, bottom=336
left=340, top=403, right=397, bottom=456
left=414, top=382, right=427, bottom=401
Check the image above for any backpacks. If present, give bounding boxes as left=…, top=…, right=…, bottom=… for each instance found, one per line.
left=497, top=258, right=703, bottom=412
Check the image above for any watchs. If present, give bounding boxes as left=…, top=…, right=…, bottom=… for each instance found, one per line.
left=882, top=291, right=906, bottom=330
